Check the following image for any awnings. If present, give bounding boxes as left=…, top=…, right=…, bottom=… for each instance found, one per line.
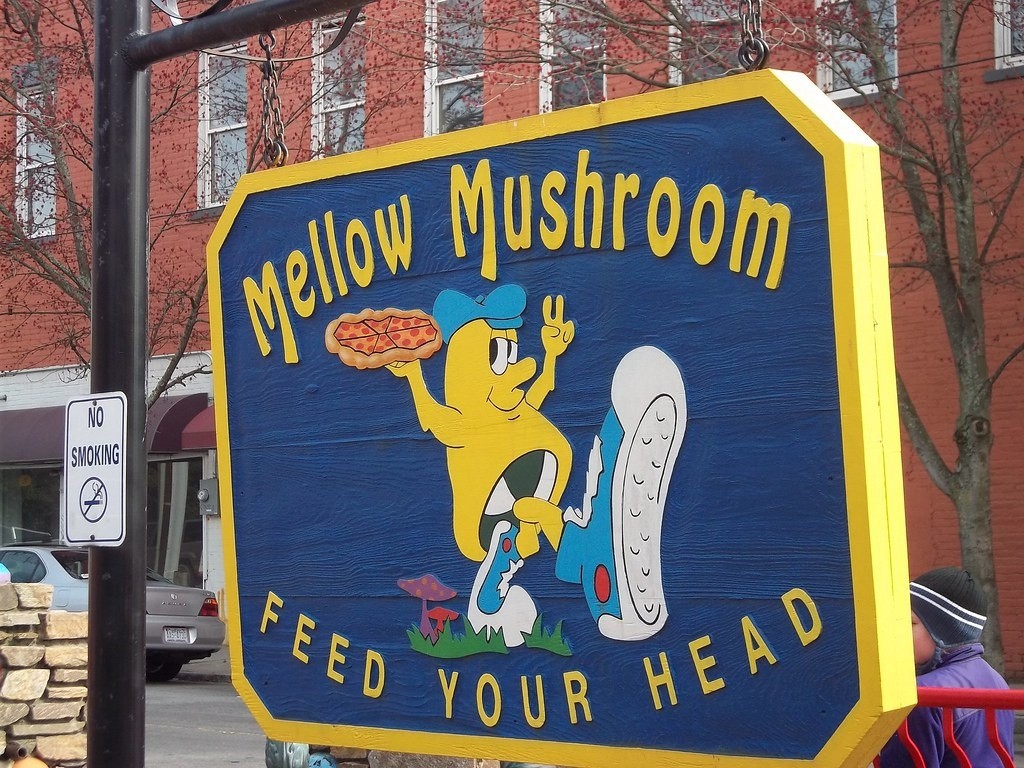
left=1, top=395, right=217, bottom=465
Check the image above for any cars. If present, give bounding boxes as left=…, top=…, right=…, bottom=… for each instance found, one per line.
left=0, top=539, right=229, bottom=684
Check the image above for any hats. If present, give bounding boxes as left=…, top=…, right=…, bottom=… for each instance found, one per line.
left=909, top=566, right=988, bottom=675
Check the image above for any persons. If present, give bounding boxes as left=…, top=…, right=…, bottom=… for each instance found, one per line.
left=878, top=564, right=1017, bottom=768
